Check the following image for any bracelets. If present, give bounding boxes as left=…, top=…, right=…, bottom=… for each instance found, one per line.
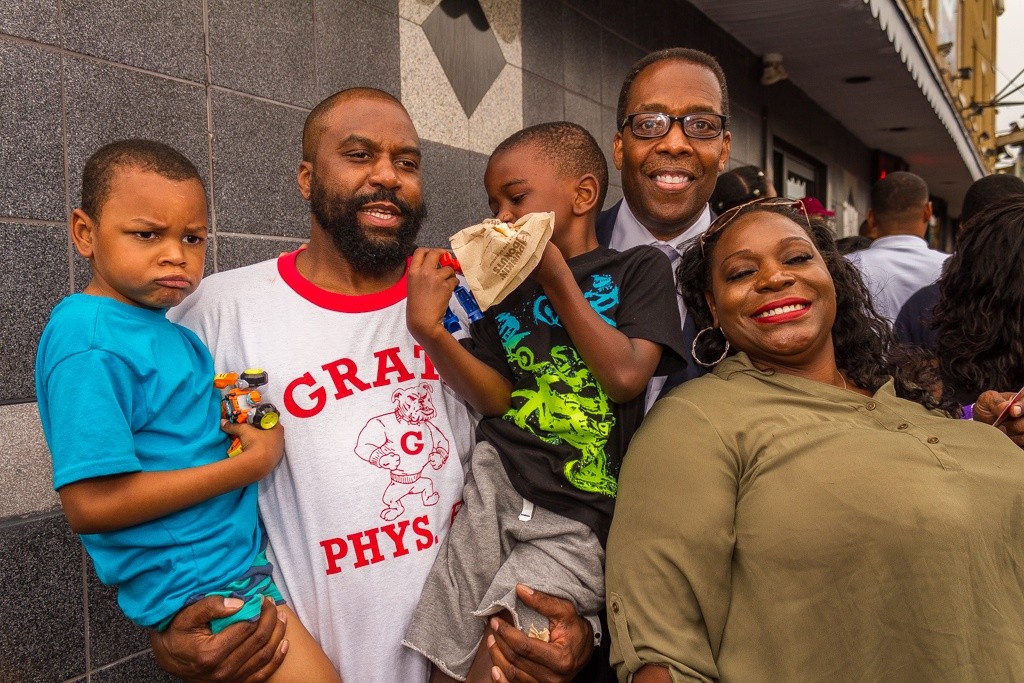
left=584, top=615, right=601, bottom=648
left=960, top=402, right=976, bottom=422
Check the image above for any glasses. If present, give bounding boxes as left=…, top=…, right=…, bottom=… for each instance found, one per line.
left=699, top=196, right=811, bottom=261
left=620, top=113, right=730, bottom=141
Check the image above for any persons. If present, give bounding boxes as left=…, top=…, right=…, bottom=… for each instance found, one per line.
left=34, top=138, right=346, bottom=683
left=604, top=196, right=1023, bottom=683
left=398, top=122, right=690, bottom=682
left=598, top=48, right=733, bottom=392
left=713, top=161, right=1024, bottom=404
left=143, top=88, right=615, bottom=683
